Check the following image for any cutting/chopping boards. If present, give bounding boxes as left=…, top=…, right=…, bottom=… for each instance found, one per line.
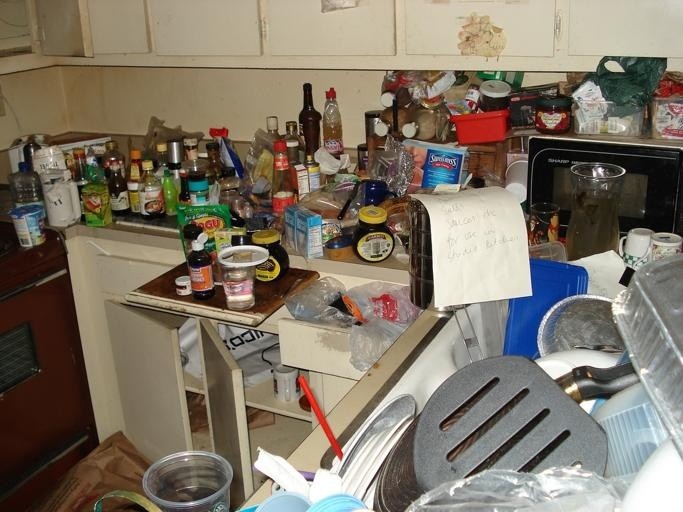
left=124, top=256, right=320, bottom=327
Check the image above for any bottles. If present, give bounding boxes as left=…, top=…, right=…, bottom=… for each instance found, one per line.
left=287, top=141, right=300, bottom=204
left=304, top=155, right=320, bottom=192
left=352, top=206, right=395, bottom=263
left=72, top=149, right=92, bottom=221
left=282, top=121, right=304, bottom=150
left=138, top=160, right=165, bottom=220
left=10, top=162, right=42, bottom=208
left=107, top=160, right=130, bottom=217
left=162, top=170, right=178, bottom=216
left=299, top=82, right=322, bottom=156
left=251, top=230, right=289, bottom=283
left=23, top=142, right=41, bottom=162
left=265, top=115, right=280, bottom=141
left=126, top=150, right=143, bottom=216
left=102, top=140, right=125, bottom=181
left=222, top=270, right=256, bottom=311
left=219, top=167, right=241, bottom=208
left=187, top=170, right=209, bottom=205
left=205, top=142, right=223, bottom=180
left=271, top=141, right=294, bottom=223
left=188, top=234, right=216, bottom=301
left=156, top=142, right=167, bottom=175
left=479, top=80, right=511, bottom=113
left=535, top=96, right=571, bottom=134
left=323, top=87, right=344, bottom=160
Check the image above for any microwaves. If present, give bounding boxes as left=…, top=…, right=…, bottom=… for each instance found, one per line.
left=526, top=118, right=683, bottom=240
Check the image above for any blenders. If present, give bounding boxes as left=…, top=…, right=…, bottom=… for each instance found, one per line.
left=42, top=168, right=81, bottom=228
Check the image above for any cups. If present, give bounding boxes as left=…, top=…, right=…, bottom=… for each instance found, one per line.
left=307, top=494, right=368, bottom=512
left=505, top=160, right=528, bottom=204
left=361, top=179, right=397, bottom=208
left=256, top=492, right=310, bottom=510
left=618, top=227, right=653, bottom=271
left=633, top=232, right=682, bottom=270
left=221, top=266, right=254, bottom=310
left=274, top=364, right=301, bottom=403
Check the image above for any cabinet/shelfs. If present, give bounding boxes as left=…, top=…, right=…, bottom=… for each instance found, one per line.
left=264, top=0, right=559, bottom=75
left=0, top=224, right=103, bottom=480
left=559, top=2, right=654, bottom=74
left=1, top=1, right=265, bottom=77
left=63, top=167, right=446, bottom=465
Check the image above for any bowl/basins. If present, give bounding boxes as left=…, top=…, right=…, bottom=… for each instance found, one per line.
left=538, top=295, right=627, bottom=357
